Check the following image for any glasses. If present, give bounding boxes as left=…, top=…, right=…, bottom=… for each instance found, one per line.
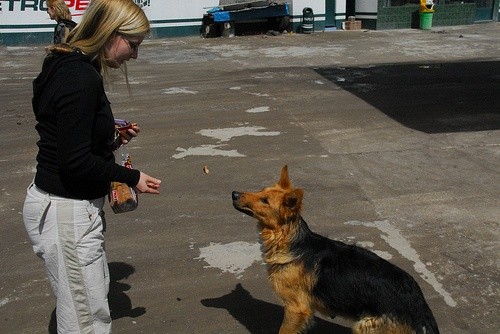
left=120, top=34, right=140, bottom=50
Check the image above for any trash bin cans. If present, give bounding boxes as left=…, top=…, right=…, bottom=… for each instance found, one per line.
left=418, top=0, right=435, bottom=29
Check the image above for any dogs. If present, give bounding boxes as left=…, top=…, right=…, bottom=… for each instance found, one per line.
left=232, top=164, right=441, bottom=334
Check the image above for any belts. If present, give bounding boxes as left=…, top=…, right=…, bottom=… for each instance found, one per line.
left=33, top=178, right=58, bottom=221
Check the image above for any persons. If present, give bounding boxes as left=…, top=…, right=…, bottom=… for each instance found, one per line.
left=46, top=0, right=78, bottom=45
left=23, top=0, right=163, bottom=334
